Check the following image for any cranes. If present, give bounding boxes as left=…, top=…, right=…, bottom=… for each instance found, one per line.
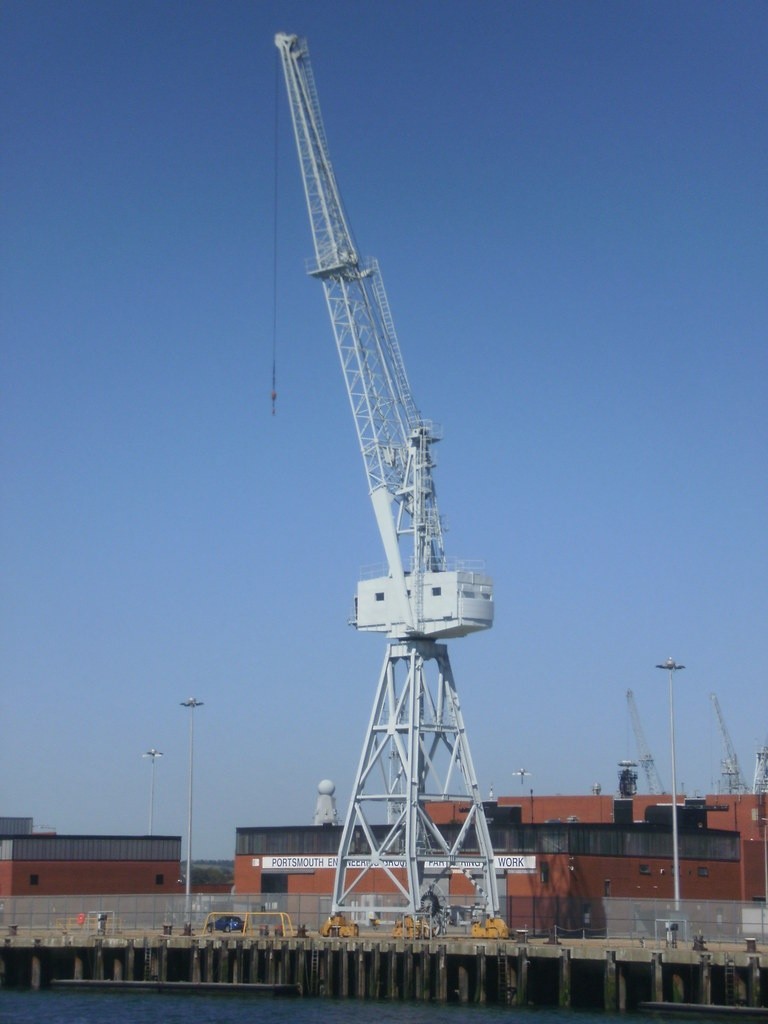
left=708, top=692, right=747, bottom=796
left=623, top=689, right=666, bottom=796
left=266, top=28, right=512, bottom=941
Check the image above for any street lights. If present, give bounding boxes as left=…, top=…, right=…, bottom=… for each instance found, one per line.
left=143, top=749, right=165, bottom=835
left=180, top=698, right=205, bottom=936
left=655, top=656, right=687, bottom=913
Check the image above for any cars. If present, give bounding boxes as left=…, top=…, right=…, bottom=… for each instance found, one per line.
left=205, top=915, right=245, bottom=932
